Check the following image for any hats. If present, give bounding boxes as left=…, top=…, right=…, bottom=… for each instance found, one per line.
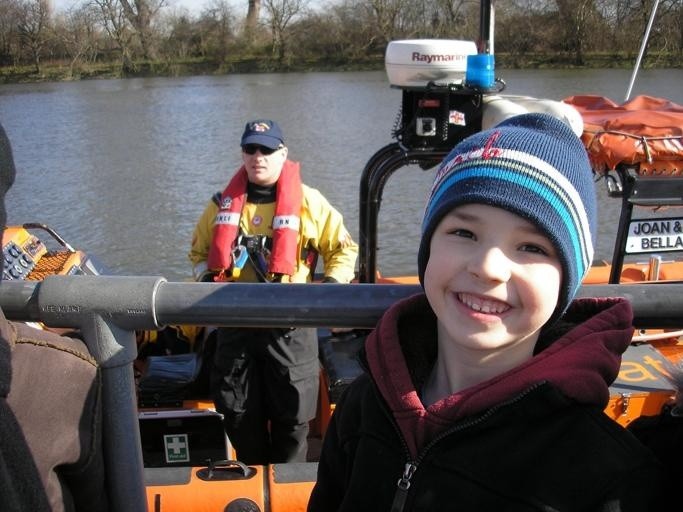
left=240, top=118, right=284, bottom=149
left=418, top=112, right=597, bottom=335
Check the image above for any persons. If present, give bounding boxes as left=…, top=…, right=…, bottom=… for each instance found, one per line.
left=189, top=121, right=357, bottom=466
left=626, top=370, right=681, bottom=512
left=1, top=127, right=110, bottom=512
left=311, top=113, right=652, bottom=512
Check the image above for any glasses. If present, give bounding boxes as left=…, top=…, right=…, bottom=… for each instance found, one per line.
left=244, top=145, right=274, bottom=155
